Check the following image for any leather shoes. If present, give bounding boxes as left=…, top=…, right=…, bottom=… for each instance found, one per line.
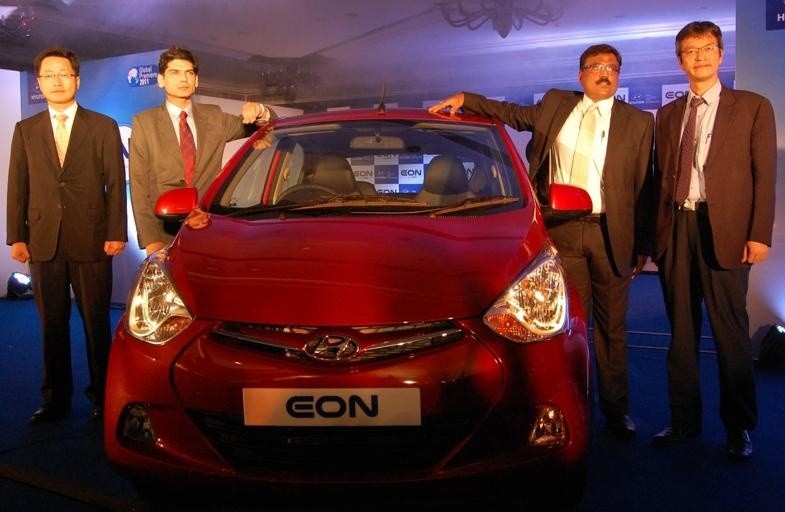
left=92, top=407, right=103, bottom=417
left=613, top=410, right=638, bottom=442
left=30, top=407, right=72, bottom=424
left=728, top=427, right=753, bottom=463
left=654, top=428, right=700, bottom=445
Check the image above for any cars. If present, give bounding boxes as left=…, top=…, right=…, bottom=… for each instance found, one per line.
left=102, top=80, right=595, bottom=490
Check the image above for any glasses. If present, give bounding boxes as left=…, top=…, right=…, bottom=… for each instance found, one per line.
left=582, top=64, right=620, bottom=73
left=678, top=44, right=719, bottom=56
left=38, top=71, right=76, bottom=83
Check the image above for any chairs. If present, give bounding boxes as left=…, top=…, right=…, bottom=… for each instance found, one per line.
left=415, top=153, right=478, bottom=205
left=311, top=154, right=362, bottom=199
left=357, top=181, right=377, bottom=197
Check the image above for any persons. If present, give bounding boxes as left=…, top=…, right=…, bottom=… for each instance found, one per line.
left=428, top=43, right=656, bottom=442
left=130, top=46, right=280, bottom=257
left=649, top=21, right=777, bottom=460
left=5, top=48, right=129, bottom=432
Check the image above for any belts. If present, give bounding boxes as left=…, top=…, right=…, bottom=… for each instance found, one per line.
left=678, top=201, right=707, bottom=213
left=577, top=212, right=606, bottom=224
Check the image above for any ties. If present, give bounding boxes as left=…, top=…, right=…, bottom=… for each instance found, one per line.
left=569, top=105, right=598, bottom=197
left=178, top=111, right=196, bottom=188
left=673, top=97, right=704, bottom=206
left=54, top=115, right=69, bottom=169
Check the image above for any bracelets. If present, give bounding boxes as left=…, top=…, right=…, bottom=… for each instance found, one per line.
left=258, top=103, right=266, bottom=118
